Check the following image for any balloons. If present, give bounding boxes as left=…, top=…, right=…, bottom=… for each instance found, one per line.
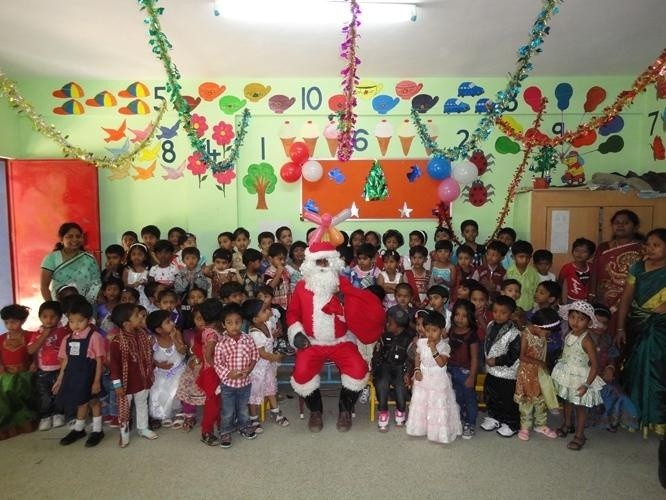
left=290, top=142, right=309, bottom=166
left=281, top=162, right=302, bottom=182
left=302, top=160, right=324, bottom=182
left=452, top=161, right=478, bottom=186
left=427, top=157, right=450, bottom=180
left=438, top=176, right=460, bottom=203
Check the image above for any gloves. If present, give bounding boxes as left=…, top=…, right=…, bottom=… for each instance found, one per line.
left=294, top=332, right=309, bottom=349
left=336, top=291, right=344, bottom=304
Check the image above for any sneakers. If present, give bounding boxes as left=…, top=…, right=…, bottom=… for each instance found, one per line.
left=39, top=413, right=196, bottom=447
left=480, top=417, right=499, bottom=431
left=462, top=423, right=474, bottom=439
left=518, top=429, right=529, bottom=440
left=337, top=410, right=352, bottom=433
left=201, top=408, right=289, bottom=447
left=309, top=410, right=322, bottom=432
left=533, top=426, right=557, bottom=438
left=378, top=411, right=390, bottom=430
left=395, top=409, right=406, bottom=426
left=497, top=423, right=518, bottom=437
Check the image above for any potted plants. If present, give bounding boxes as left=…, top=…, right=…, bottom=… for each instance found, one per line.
left=528, top=142, right=561, bottom=188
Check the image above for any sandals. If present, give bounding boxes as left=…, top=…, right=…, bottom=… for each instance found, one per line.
left=557, top=425, right=575, bottom=437
left=567, top=435, right=585, bottom=450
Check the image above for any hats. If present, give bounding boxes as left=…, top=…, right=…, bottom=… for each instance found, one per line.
left=558, top=299, right=605, bottom=329
left=305, top=241, right=337, bottom=260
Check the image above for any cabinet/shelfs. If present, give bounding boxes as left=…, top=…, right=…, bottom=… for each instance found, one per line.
left=512, top=185, right=666, bottom=281
